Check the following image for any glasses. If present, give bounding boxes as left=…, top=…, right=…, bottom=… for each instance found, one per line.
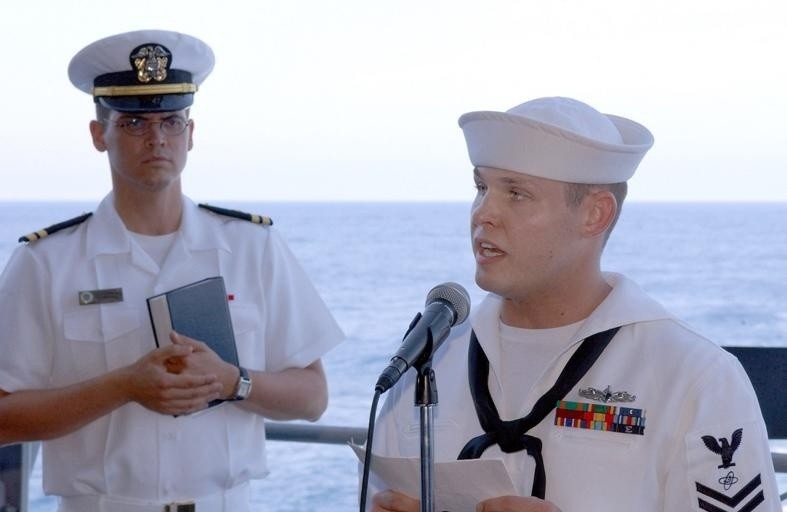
left=105, top=116, right=191, bottom=135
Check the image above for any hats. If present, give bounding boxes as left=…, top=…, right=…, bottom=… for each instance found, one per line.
left=458, top=97, right=654, bottom=183
left=67, top=29, right=215, bottom=111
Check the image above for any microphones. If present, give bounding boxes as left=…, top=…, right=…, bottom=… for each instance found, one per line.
left=372, top=280, right=471, bottom=397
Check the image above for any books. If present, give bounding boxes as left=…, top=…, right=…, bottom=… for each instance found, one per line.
left=146, top=277, right=239, bottom=418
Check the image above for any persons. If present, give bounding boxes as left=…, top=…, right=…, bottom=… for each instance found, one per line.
left=359, top=97, right=783, bottom=512
left=1, top=31, right=347, bottom=512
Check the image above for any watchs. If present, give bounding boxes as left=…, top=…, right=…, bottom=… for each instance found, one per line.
left=232, top=366, right=252, bottom=400
